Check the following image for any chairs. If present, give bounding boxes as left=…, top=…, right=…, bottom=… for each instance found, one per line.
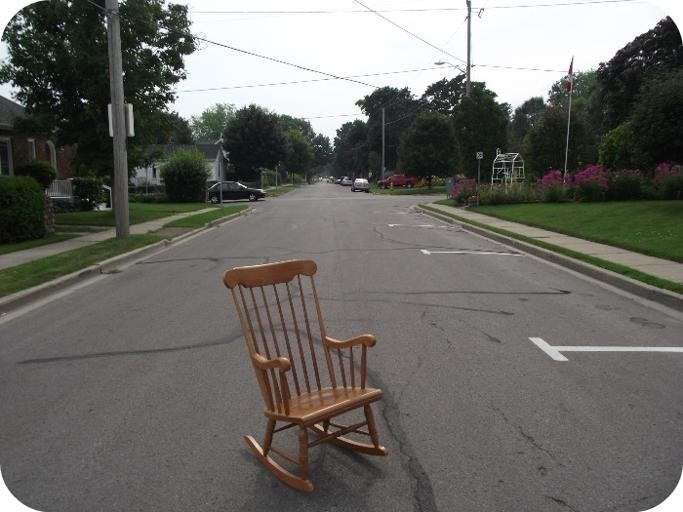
left=222, top=259, right=388, bottom=493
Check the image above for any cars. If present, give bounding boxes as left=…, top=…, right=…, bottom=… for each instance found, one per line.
left=376, top=174, right=418, bottom=189
left=350, top=178, right=368, bottom=192
left=334, top=176, right=353, bottom=186
left=207, top=180, right=265, bottom=204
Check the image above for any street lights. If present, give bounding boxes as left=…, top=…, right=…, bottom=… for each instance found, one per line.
left=434, top=0, right=470, bottom=98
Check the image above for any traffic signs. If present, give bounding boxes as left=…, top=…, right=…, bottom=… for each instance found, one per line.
left=475, top=151, right=483, bottom=159
